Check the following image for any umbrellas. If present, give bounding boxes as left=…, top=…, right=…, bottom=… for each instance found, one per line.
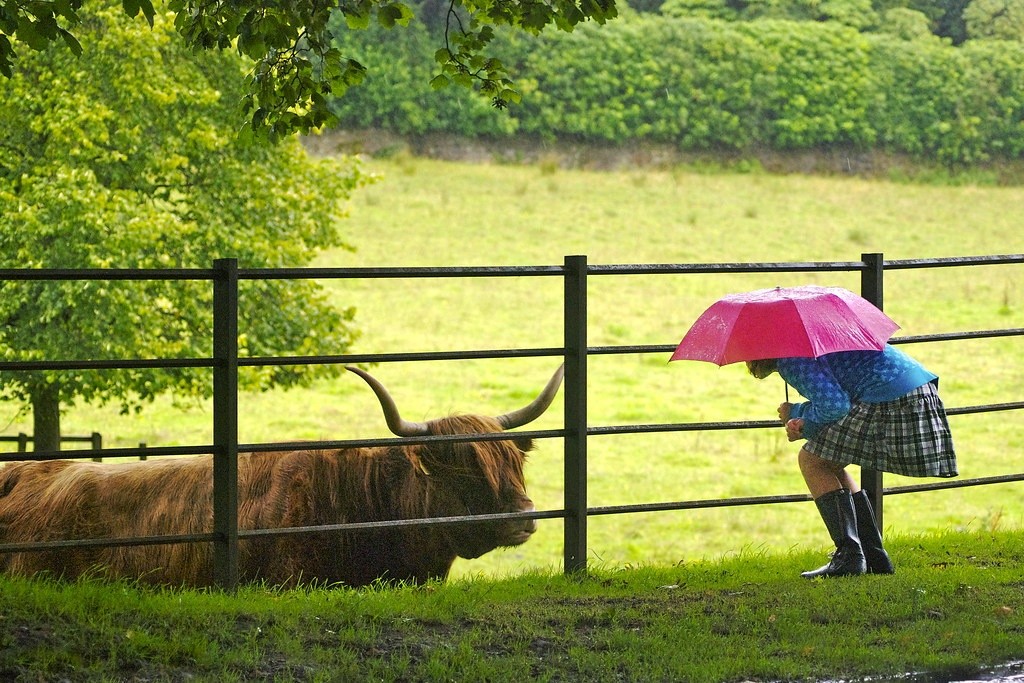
left=667, top=286, right=902, bottom=403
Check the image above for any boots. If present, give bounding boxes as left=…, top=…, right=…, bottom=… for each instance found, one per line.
left=801, top=488, right=867, bottom=578
left=852, top=489, right=894, bottom=574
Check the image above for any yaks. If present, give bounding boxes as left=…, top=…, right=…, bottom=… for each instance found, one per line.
left=0, top=362, right=566, bottom=583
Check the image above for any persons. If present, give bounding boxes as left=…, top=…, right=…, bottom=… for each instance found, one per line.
left=744, top=342, right=959, bottom=579
